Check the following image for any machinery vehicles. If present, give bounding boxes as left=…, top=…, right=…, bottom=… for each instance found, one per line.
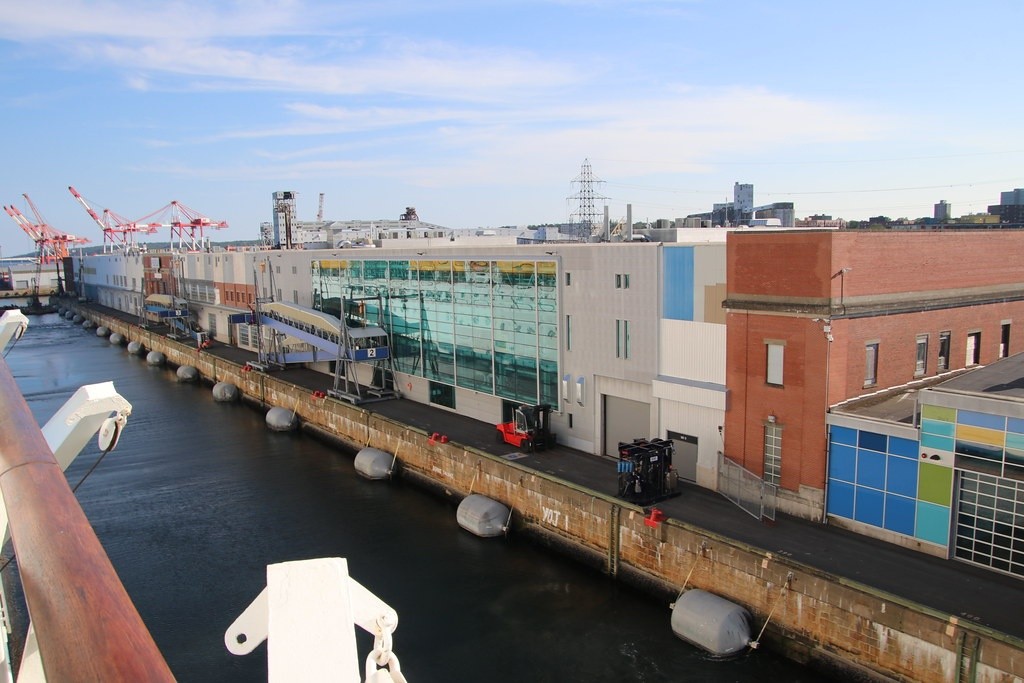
left=495, top=403, right=558, bottom=455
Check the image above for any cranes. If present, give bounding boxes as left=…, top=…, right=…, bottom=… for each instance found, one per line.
left=67, top=185, right=228, bottom=251
left=3, top=192, right=93, bottom=265
